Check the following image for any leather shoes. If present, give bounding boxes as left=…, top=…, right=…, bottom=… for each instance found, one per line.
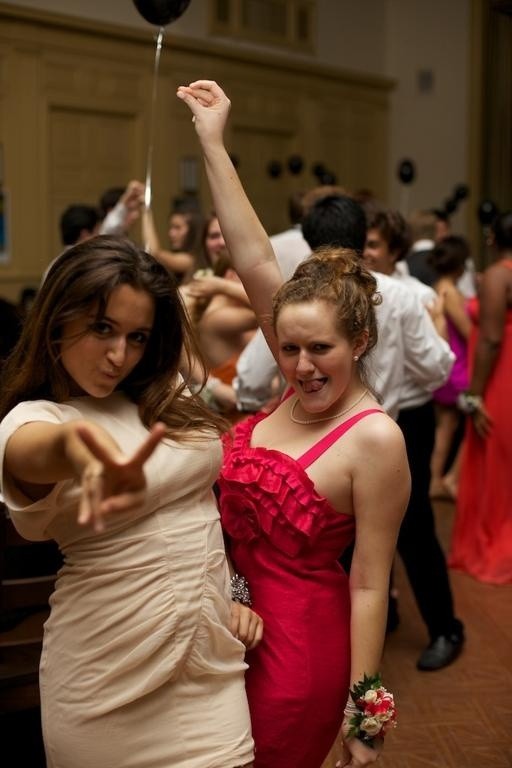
left=385, top=606, right=401, bottom=636
left=416, top=621, right=468, bottom=673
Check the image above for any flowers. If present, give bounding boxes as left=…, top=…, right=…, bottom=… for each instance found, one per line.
left=344, top=670, right=399, bottom=747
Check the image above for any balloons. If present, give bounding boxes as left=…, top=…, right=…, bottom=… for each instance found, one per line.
left=133, top=0, right=190, bottom=25
